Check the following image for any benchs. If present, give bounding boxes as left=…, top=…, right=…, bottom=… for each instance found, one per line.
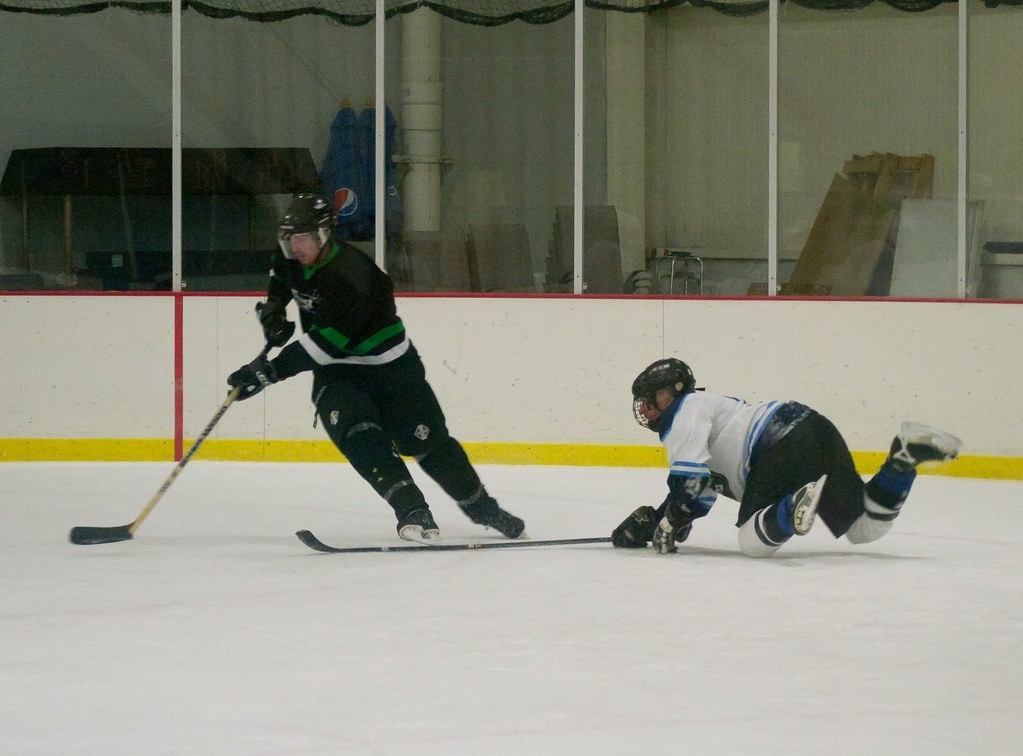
left=84, top=248, right=270, bottom=291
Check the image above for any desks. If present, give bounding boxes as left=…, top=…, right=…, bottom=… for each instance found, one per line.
left=0, top=147, right=321, bottom=289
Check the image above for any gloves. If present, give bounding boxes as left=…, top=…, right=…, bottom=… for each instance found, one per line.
left=227, top=361, right=283, bottom=401
left=651, top=503, right=698, bottom=553
left=611, top=506, right=656, bottom=548
left=254, top=300, right=296, bottom=347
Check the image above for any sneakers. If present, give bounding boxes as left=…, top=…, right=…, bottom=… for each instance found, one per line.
left=886, top=423, right=960, bottom=475
left=790, top=473, right=828, bottom=536
left=457, top=485, right=528, bottom=539
left=396, top=507, right=443, bottom=547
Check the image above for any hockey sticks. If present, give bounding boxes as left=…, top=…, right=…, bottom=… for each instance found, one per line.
left=294, top=528, right=616, bottom=554
left=67, top=314, right=286, bottom=547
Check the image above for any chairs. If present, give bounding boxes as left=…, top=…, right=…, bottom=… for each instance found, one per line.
left=465, top=224, right=534, bottom=292
left=624, top=269, right=660, bottom=295
left=390, top=230, right=471, bottom=292
left=542, top=204, right=624, bottom=295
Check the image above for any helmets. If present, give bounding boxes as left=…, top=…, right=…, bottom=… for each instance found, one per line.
left=631, top=358, right=695, bottom=432
left=280, top=192, right=338, bottom=234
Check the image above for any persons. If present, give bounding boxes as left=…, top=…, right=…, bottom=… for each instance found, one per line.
left=226, top=194, right=525, bottom=544
left=609, top=357, right=958, bottom=558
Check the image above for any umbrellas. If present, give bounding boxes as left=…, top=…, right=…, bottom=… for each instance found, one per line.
left=315, top=94, right=404, bottom=241
